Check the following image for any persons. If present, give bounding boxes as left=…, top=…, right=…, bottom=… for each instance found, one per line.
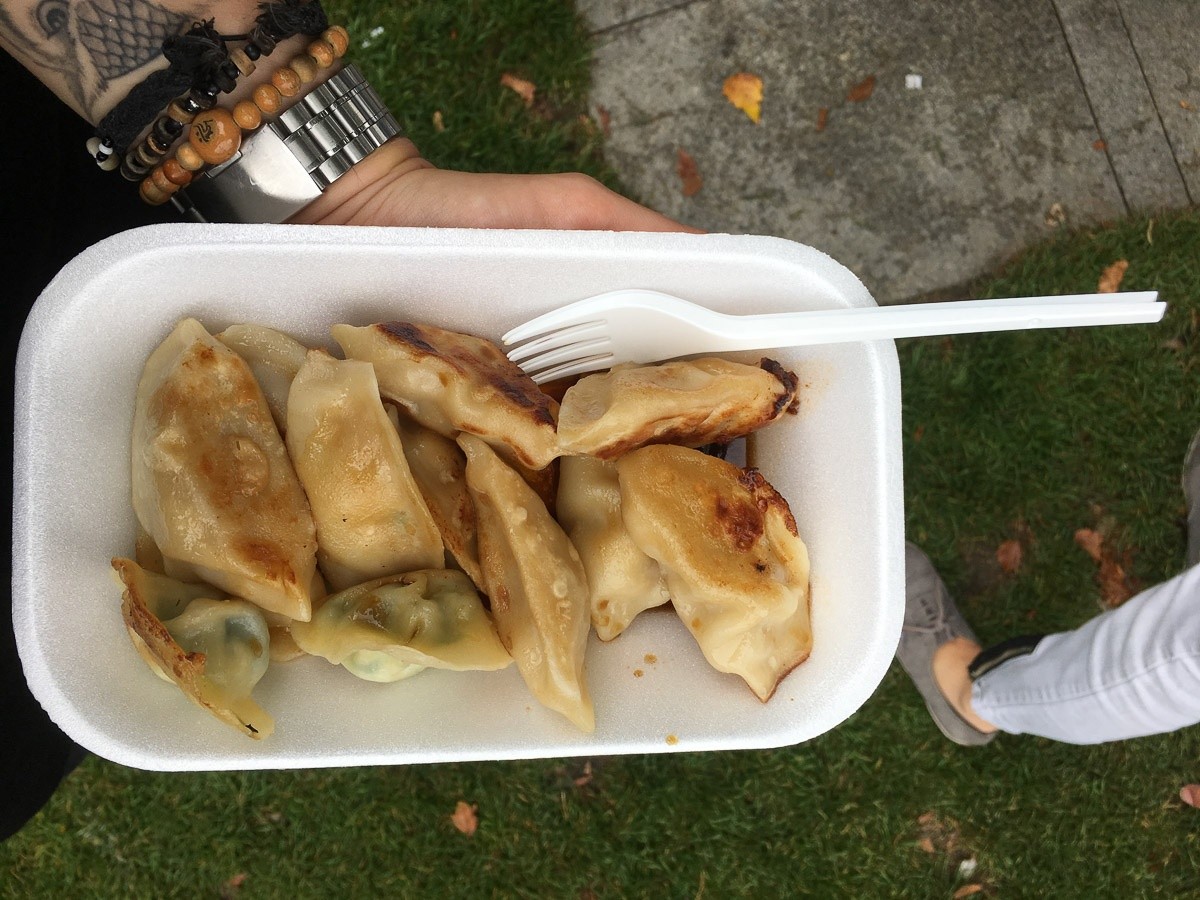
left=0, top=0, right=710, bottom=837
left=891, top=436, right=1200, bottom=810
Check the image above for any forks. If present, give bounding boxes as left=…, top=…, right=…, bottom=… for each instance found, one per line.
left=498, top=288, right=1166, bottom=384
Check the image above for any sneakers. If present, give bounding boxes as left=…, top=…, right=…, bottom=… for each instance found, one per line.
left=1184, top=426, right=1200, bottom=568
left=898, top=537, right=1001, bottom=748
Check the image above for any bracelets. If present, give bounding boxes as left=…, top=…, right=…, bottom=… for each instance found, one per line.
left=86, top=1, right=404, bottom=224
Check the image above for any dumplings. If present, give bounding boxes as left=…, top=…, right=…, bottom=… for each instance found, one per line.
left=108, top=320, right=812, bottom=739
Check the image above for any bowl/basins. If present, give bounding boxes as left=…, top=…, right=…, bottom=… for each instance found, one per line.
left=11, top=223, right=906, bottom=772
